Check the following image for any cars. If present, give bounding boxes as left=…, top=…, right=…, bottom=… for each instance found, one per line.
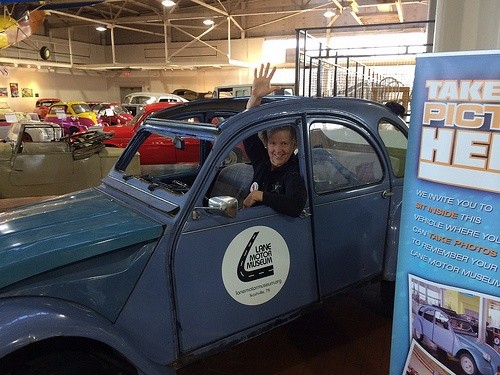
left=414, top=304, right=500, bottom=374
left=0, top=88, right=200, bottom=142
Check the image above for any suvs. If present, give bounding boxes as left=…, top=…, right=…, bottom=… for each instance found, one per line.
left=102, top=102, right=249, bottom=184
left=0, top=95, right=410, bottom=375
left=0, top=120, right=141, bottom=200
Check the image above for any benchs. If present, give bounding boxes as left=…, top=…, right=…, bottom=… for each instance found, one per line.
left=312, top=148, right=400, bottom=191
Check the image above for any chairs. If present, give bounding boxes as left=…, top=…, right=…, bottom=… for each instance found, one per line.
left=210, top=162, right=255, bottom=204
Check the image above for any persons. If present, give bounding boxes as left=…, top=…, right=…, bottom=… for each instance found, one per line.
left=241, top=61, right=308, bottom=219
